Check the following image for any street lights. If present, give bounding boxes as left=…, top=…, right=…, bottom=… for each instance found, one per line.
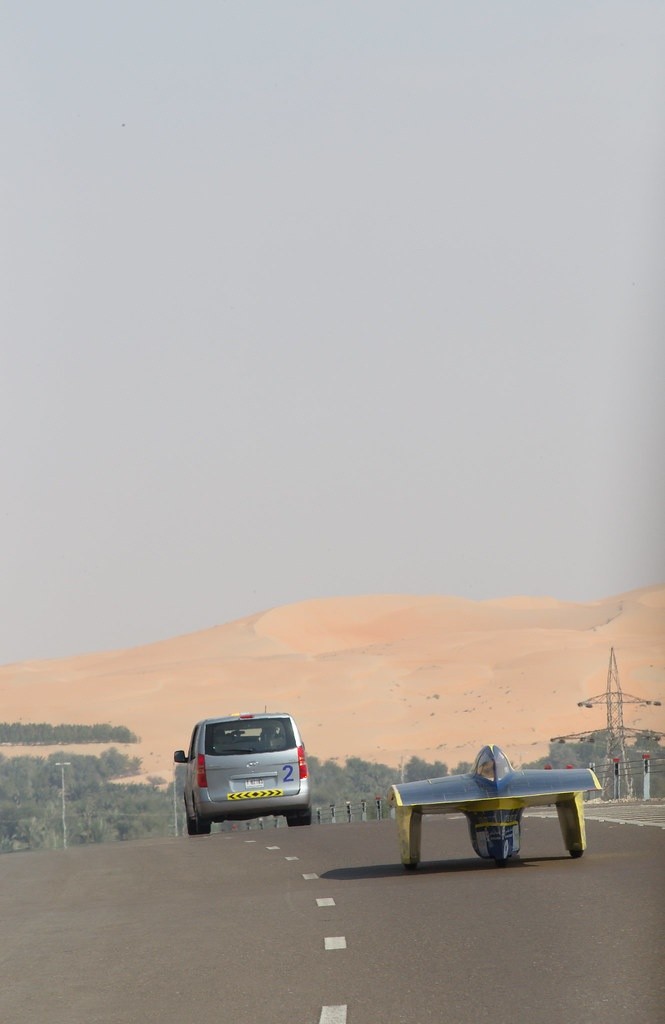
left=54, top=761, right=70, bottom=849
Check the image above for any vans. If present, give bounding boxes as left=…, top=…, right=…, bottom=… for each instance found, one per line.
left=174, top=714, right=312, bottom=836
